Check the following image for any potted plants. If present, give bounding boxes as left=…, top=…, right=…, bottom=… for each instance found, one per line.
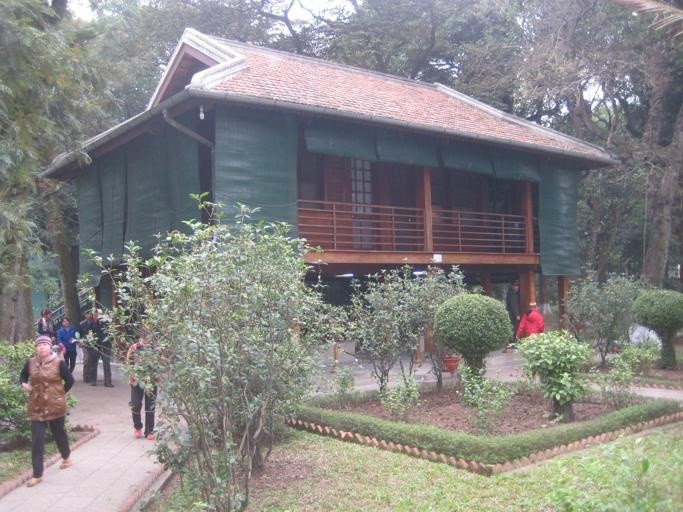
left=441, top=346, right=459, bottom=370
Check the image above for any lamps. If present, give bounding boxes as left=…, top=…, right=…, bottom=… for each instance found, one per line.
left=197, top=104, right=205, bottom=119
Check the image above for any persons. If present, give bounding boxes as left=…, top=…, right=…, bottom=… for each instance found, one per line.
left=506, top=280, right=521, bottom=342
left=20, top=306, right=162, bottom=488
left=515, top=302, right=545, bottom=338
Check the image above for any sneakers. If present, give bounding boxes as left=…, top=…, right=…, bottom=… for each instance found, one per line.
left=145, top=433, right=154, bottom=440
left=25, top=474, right=42, bottom=488
left=132, top=428, right=142, bottom=438
left=58, top=457, right=72, bottom=469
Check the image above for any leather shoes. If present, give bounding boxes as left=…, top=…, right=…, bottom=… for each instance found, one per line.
left=91, top=381, right=114, bottom=388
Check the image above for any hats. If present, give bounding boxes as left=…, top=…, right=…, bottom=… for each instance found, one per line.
left=33, top=334, right=52, bottom=347
